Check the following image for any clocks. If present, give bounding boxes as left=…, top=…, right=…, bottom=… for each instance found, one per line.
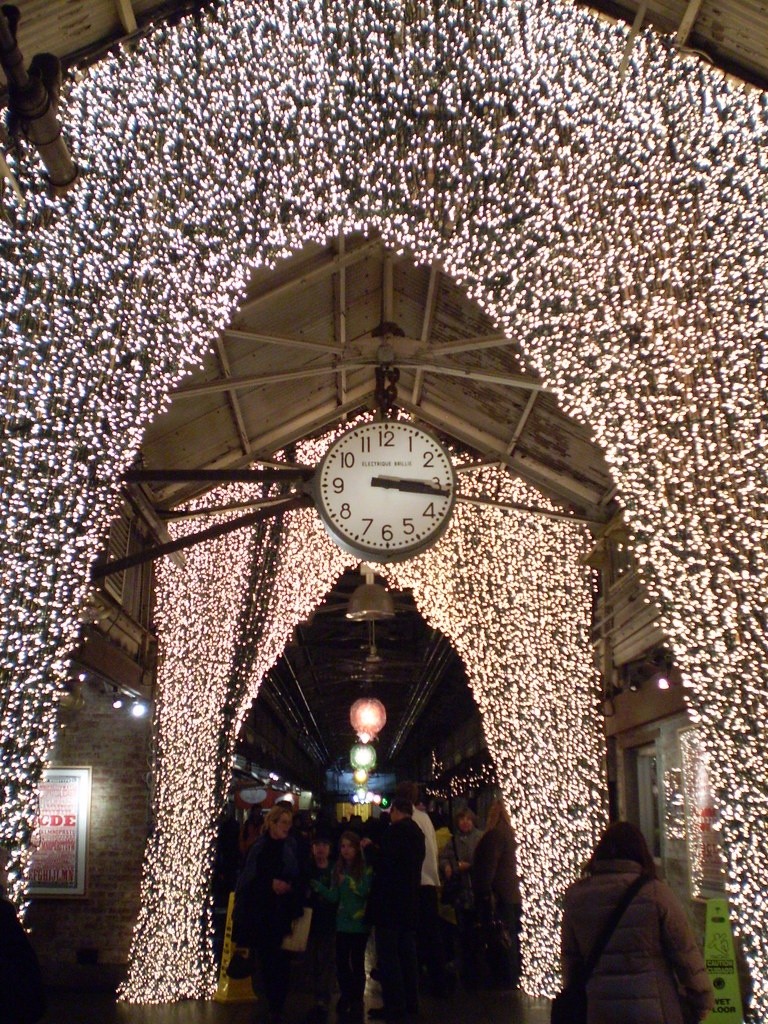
left=317, top=420, right=455, bottom=563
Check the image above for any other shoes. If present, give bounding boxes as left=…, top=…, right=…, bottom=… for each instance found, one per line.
left=367, top=1005, right=418, bottom=1020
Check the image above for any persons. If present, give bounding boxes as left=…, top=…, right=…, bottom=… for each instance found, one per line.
left=560, top=821, right=715, bottom=1024
left=229, top=781, right=520, bottom=1024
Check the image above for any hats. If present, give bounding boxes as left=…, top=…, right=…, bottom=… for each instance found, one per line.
left=393, top=798, right=413, bottom=815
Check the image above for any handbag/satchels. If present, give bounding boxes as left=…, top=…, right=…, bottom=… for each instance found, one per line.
left=551, top=978, right=586, bottom=1024
left=225, top=948, right=260, bottom=978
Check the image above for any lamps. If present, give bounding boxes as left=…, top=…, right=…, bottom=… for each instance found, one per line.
left=342, top=581, right=396, bottom=663
left=347, top=690, right=391, bottom=784
left=130, top=700, right=144, bottom=718
left=113, top=695, right=122, bottom=710
left=630, top=679, right=641, bottom=691
